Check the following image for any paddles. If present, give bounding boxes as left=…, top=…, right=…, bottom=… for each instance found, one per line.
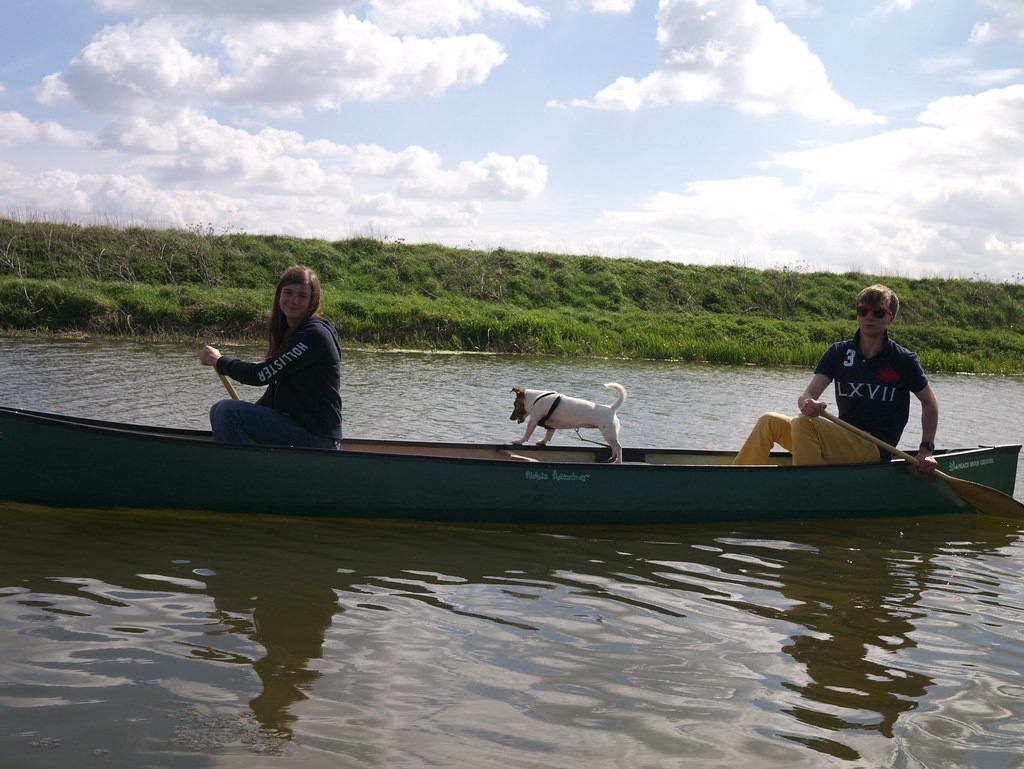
left=821, top=409, right=1024, bottom=521
left=214, top=363, right=241, bottom=401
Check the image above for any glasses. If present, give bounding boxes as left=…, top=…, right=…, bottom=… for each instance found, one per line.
left=857, top=306, right=893, bottom=319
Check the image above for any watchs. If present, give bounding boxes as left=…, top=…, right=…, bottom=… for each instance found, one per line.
left=919, top=442, right=935, bottom=452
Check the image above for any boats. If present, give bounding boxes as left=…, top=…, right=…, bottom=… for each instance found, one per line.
left=0, top=403, right=1023, bottom=529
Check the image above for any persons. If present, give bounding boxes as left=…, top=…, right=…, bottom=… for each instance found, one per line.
left=732, top=285, right=939, bottom=476
left=200, top=263, right=344, bottom=452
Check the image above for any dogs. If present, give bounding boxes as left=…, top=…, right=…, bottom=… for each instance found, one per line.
left=509, top=382, right=627, bottom=464
